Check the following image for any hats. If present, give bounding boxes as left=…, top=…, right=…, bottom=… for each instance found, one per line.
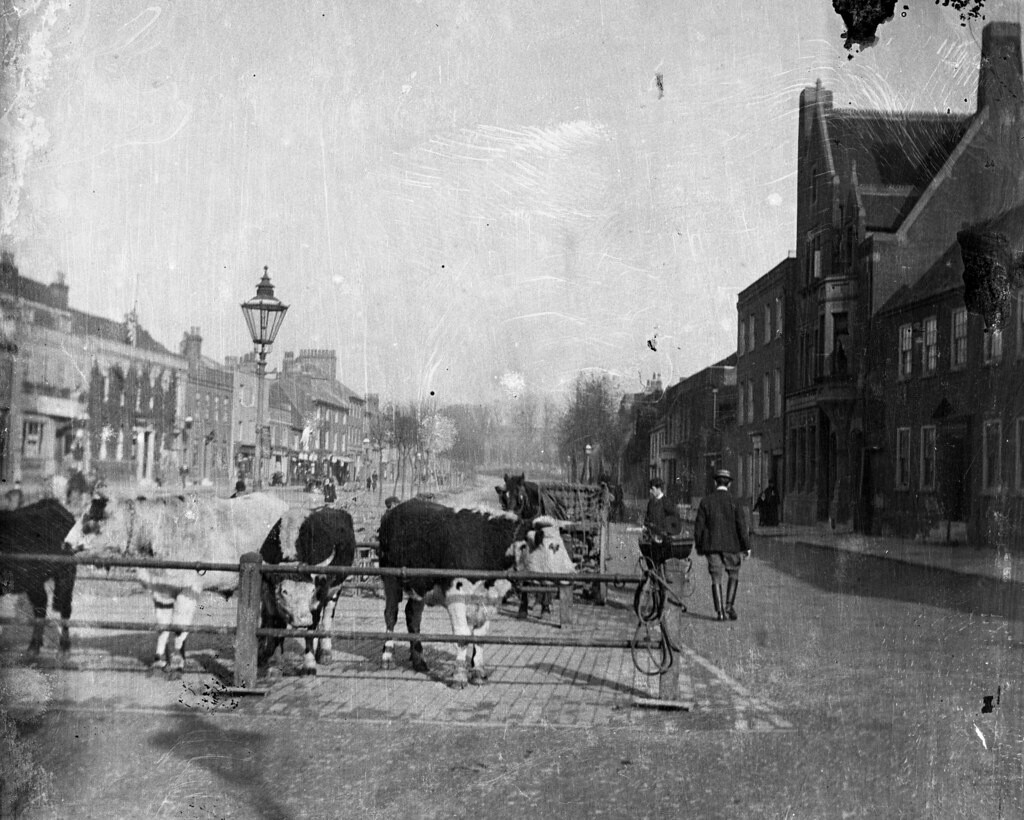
left=649, top=478, right=665, bottom=488
left=712, top=468, right=734, bottom=481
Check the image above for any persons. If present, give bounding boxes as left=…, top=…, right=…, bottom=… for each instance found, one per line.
left=366, top=477, right=371, bottom=489
left=5, top=479, right=25, bottom=510
left=752, top=492, right=767, bottom=526
left=694, top=470, right=750, bottom=620
left=235, top=476, right=245, bottom=492
left=644, top=479, right=678, bottom=583
left=765, top=479, right=781, bottom=526
left=372, top=471, right=378, bottom=492
left=601, top=470, right=611, bottom=492
left=324, top=477, right=337, bottom=502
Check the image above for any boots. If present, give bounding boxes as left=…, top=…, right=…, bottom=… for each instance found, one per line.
left=712, top=583, right=726, bottom=621
left=726, top=579, right=738, bottom=620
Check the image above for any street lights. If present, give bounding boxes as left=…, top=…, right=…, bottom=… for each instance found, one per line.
left=240, top=266, right=292, bottom=492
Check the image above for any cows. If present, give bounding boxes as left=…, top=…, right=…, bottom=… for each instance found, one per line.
left=0, top=490, right=357, bottom=674
left=377, top=497, right=575, bottom=689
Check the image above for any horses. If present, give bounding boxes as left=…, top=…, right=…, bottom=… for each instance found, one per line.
left=494, top=473, right=624, bottom=525
